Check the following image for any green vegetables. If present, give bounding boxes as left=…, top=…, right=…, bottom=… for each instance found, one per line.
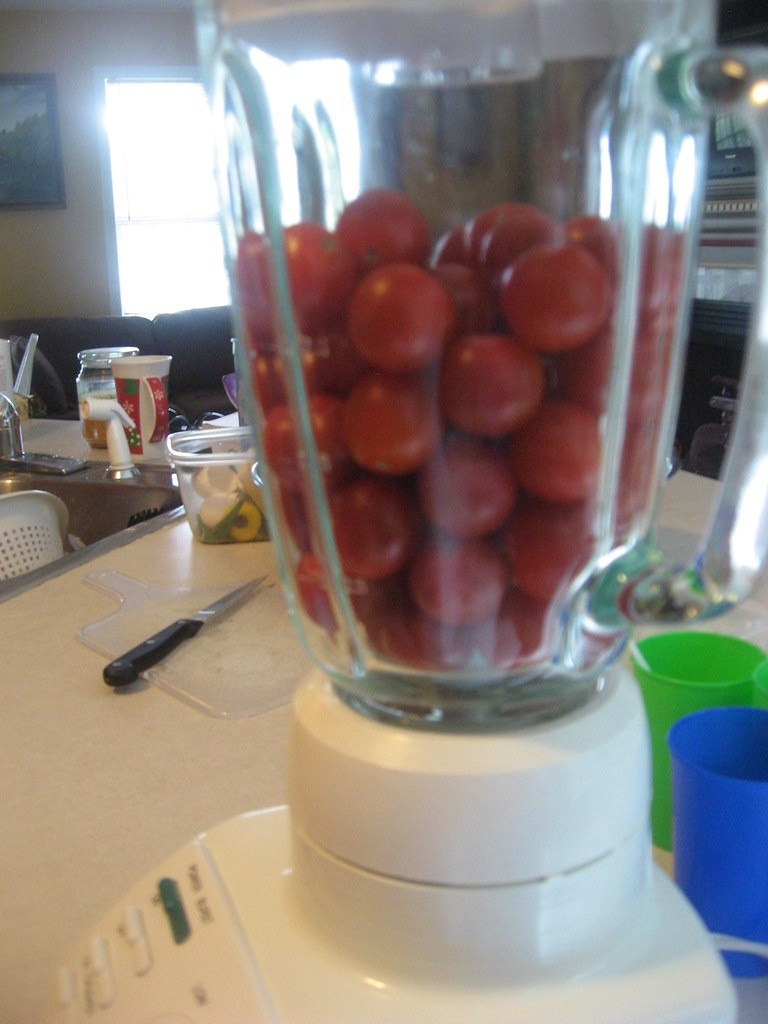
left=193, top=485, right=269, bottom=545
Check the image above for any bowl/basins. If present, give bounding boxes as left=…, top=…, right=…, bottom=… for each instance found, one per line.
left=163, top=426, right=272, bottom=545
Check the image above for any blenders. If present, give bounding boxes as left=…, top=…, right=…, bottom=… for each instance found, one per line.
left=29, top=0, right=744, bottom=1024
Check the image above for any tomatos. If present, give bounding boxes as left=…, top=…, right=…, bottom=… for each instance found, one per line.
left=234, top=186, right=691, bottom=679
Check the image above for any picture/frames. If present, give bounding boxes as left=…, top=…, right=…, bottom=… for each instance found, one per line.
left=0, top=71, right=66, bottom=213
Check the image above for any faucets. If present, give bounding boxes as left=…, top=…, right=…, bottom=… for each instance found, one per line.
left=0, top=393, right=27, bottom=459
left=81, top=397, right=142, bottom=480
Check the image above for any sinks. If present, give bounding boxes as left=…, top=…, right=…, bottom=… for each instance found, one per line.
left=0, top=458, right=191, bottom=604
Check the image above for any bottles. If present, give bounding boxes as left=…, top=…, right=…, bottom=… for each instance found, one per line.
left=75, top=347, right=140, bottom=449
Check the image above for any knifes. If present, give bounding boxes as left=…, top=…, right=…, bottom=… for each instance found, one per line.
left=103, top=574, right=269, bottom=688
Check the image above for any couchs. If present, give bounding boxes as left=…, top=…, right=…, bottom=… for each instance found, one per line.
left=0, top=305, right=238, bottom=425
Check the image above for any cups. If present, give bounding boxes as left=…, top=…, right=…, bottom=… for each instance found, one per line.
left=111, top=356, right=173, bottom=461
left=630, top=631, right=768, bottom=853
left=668, top=705, right=768, bottom=979
